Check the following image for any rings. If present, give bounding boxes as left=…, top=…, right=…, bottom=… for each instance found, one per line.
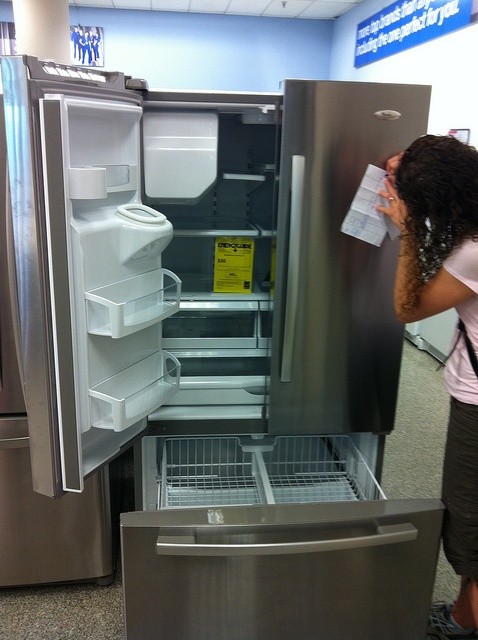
left=388, top=196, right=395, bottom=202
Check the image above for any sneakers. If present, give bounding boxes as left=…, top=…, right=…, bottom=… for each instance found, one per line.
left=428, top=600, right=478, bottom=639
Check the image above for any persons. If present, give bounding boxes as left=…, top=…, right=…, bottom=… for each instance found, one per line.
left=375, top=135, right=478, bottom=638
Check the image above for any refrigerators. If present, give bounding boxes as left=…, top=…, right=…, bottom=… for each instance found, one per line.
left=0, top=89, right=113, bottom=586
left=1, top=54, right=447, bottom=639
